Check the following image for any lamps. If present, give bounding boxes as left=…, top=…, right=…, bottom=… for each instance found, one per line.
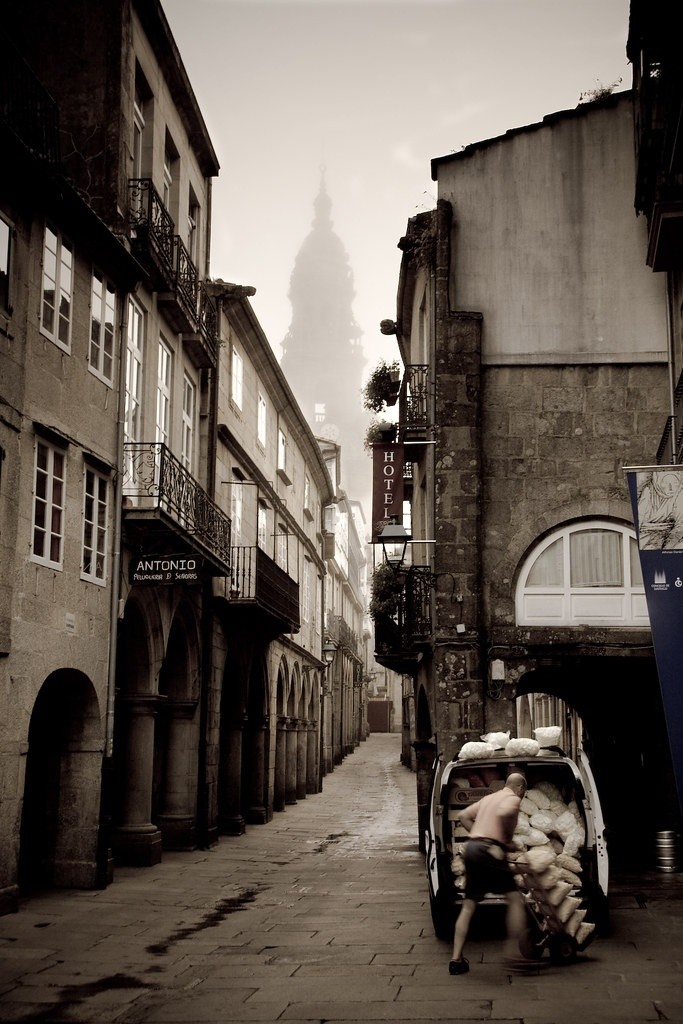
left=487, top=655, right=506, bottom=699
left=377, top=513, right=432, bottom=589
left=303, top=640, right=338, bottom=672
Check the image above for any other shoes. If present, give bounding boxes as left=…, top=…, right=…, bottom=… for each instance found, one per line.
left=449, top=957, right=469, bottom=975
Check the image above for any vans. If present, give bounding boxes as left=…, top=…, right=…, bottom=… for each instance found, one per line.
left=426, top=746, right=610, bottom=942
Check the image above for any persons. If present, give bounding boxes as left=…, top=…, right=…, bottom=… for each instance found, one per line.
left=448, top=772, right=529, bottom=975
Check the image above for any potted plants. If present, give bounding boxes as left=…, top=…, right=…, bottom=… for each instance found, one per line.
left=362, top=422, right=392, bottom=447
left=371, top=560, right=407, bottom=620
left=364, top=363, right=401, bottom=415
